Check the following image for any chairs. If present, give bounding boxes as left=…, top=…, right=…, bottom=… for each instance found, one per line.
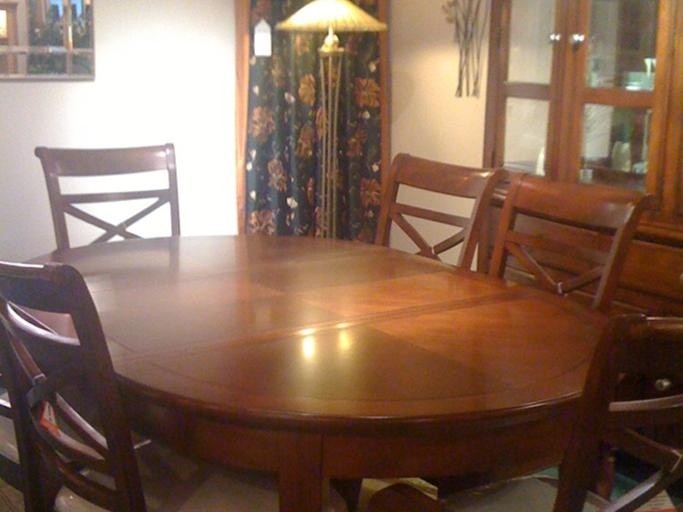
left=31, top=142, right=187, bottom=250
left=375, top=152, right=508, bottom=277
left=2, top=257, right=155, bottom=509
left=459, top=315, right=682, bottom=512
left=488, top=173, right=653, bottom=313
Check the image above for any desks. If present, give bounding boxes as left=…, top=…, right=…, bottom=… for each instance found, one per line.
left=4, top=232, right=620, bottom=507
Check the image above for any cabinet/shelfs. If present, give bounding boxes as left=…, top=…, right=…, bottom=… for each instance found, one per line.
left=475, top=0, right=683, bottom=318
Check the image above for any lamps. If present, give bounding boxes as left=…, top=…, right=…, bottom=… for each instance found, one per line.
left=274, top=0, right=389, bottom=239
